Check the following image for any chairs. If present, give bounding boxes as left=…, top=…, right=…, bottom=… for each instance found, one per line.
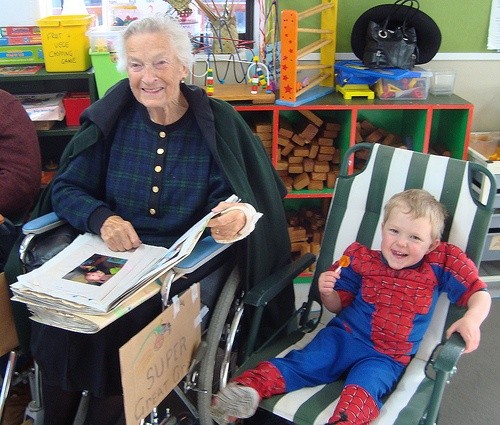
left=229, top=142, right=498, bottom=425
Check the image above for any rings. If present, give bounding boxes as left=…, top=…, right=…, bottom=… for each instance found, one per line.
left=215, top=227, right=220, bottom=235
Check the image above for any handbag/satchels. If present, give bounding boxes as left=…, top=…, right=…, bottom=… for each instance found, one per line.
left=364, top=0, right=419, bottom=68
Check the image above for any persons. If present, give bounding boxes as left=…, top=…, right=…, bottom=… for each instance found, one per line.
left=82, top=254, right=125, bottom=283
left=207, top=188, right=491, bottom=425
left=26, top=14, right=264, bottom=425
left=1, top=87, right=42, bottom=390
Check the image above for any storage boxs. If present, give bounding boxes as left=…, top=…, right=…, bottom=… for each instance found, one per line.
left=376, top=67, right=434, bottom=101
left=84, top=25, right=128, bottom=52
left=36, top=15, right=97, bottom=71
left=11, top=93, right=91, bottom=131
left=89, top=47, right=129, bottom=100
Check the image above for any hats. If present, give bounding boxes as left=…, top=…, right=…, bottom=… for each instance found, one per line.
left=351, top=4, right=441, bottom=64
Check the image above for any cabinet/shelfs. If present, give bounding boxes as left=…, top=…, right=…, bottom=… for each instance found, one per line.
left=0, top=65, right=98, bottom=189
left=231, top=94, right=500, bottom=285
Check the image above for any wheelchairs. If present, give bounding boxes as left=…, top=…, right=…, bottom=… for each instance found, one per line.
left=0, top=208, right=316, bottom=424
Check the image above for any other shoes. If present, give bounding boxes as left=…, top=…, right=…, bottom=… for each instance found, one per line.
left=210, top=382, right=259, bottom=425
